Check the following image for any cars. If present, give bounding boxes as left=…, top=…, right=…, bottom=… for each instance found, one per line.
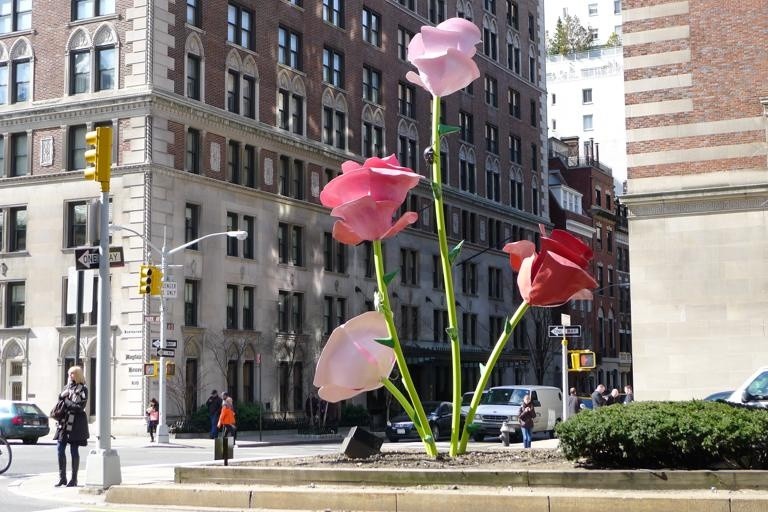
left=383, top=398, right=473, bottom=446
left=572, top=395, right=605, bottom=416
left=605, top=393, right=628, bottom=406
left=691, top=361, right=768, bottom=425
left=0, top=399, right=50, bottom=446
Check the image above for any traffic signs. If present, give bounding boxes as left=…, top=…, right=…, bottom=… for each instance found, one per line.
left=73, top=243, right=124, bottom=271
left=547, top=324, right=584, bottom=338
left=150, top=339, right=180, bottom=347
left=155, top=347, right=177, bottom=358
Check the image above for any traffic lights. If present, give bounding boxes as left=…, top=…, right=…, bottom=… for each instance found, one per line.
left=80, top=124, right=109, bottom=181
left=157, top=269, right=166, bottom=294
left=141, top=266, right=153, bottom=294
left=166, top=362, right=176, bottom=376
left=575, top=351, right=597, bottom=370
left=143, top=361, right=157, bottom=377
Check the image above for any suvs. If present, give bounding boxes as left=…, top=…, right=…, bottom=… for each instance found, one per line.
left=455, top=387, right=491, bottom=428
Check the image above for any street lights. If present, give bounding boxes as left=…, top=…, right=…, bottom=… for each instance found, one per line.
left=106, top=218, right=250, bottom=446
left=553, top=282, right=630, bottom=312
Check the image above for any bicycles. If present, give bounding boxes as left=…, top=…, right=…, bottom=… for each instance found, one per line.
left=0, top=435, right=14, bottom=477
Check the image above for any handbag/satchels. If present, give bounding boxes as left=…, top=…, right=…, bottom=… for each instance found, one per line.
left=49, top=399, right=67, bottom=421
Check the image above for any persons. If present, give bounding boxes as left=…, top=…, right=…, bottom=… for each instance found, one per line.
left=519, top=395, right=536, bottom=448
left=146, top=398, right=158, bottom=442
left=50, top=364, right=89, bottom=486
left=568, top=384, right=633, bottom=415
left=206, top=390, right=222, bottom=439
left=305, top=392, right=335, bottom=424
left=222, top=391, right=233, bottom=407
left=216, top=400, right=237, bottom=445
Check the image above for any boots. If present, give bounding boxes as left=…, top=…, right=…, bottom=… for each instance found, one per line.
left=66, top=454, right=79, bottom=487
left=54, top=454, right=67, bottom=487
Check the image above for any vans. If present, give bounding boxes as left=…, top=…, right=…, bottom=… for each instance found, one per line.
left=465, top=382, right=567, bottom=444
